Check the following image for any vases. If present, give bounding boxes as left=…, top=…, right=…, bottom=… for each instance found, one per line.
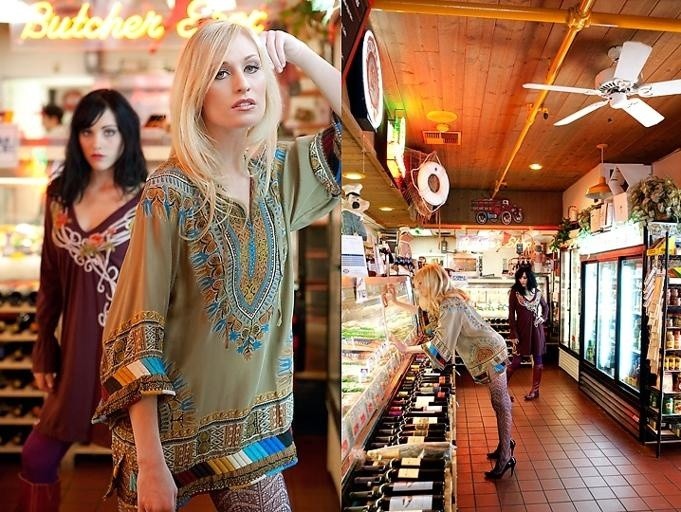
left=649, top=198, right=675, bottom=223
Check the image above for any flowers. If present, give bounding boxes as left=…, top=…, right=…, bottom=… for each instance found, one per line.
left=627, top=171, right=680, bottom=230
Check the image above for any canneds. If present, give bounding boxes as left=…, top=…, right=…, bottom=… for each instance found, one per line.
left=658, top=357, right=680, bottom=370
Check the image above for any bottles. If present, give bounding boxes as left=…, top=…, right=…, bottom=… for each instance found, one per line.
left=667, top=331, right=681, bottom=348
left=0, top=279, right=45, bottom=448
left=344, top=355, right=455, bottom=512
left=667, top=313, right=681, bottom=326
left=365, top=241, right=415, bottom=277
left=517, top=257, right=531, bottom=275
left=656, top=373, right=681, bottom=392
left=668, top=287, right=681, bottom=305
left=484, top=317, right=511, bottom=355
left=646, top=391, right=681, bottom=437
left=521, top=358, right=531, bottom=362
left=659, top=353, right=681, bottom=371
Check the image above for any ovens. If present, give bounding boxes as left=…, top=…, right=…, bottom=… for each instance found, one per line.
left=448, top=252, right=484, bottom=278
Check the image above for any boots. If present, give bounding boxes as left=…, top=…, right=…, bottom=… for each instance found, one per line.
left=525, top=365, right=543, bottom=399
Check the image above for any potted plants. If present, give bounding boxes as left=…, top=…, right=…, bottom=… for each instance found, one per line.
left=548, top=218, right=583, bottom=252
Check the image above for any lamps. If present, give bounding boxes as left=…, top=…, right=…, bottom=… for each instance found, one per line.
left=586, top=144, right=615, bottom=199
left=427, top=110, right=457, bottom=132
left=606, top=88, right=628, bottom=110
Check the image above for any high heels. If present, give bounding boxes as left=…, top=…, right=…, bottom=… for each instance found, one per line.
left=487, top=440, right=515, bottom=458
left=485, top=457, right=516, bottom=478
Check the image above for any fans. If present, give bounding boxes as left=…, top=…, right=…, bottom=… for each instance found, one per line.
left=522, top=40, right=681, bottom=127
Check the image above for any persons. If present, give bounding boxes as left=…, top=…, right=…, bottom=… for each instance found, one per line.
left=387, top=264, right=516, bottom=478
left=20, top=89, right=149, bottom=512
left=0, top=100, right=70, bottom=227
left=93, top=19, right=342, bottom=512
left=508, top=266, right=549, bottom=399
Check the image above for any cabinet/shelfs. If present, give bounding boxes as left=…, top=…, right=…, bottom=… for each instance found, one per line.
left=1, top=139, right=176, bottom=457
left=342, top=272, right=459, bottom=511
left=636, top=220, right=680, bottom=461
left=456, top=322, right=535, bottom=367
